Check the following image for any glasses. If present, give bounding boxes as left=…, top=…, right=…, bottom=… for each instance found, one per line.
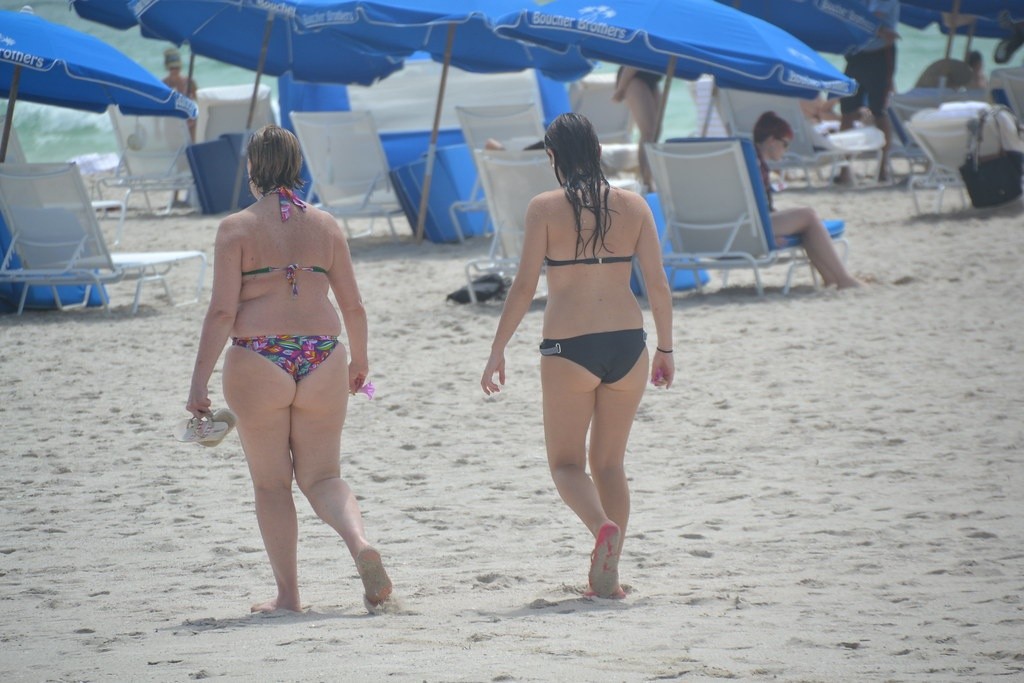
left=781, top=137, right=789, bottom=148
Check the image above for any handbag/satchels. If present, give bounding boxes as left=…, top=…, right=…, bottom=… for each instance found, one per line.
left=958, top=112, right=1024, bottom=208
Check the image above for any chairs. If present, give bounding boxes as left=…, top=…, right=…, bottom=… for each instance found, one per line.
left=0, top=63, right=1024, bottom=309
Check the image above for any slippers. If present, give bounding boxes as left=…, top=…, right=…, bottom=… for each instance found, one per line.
left=584, top=522, right=626, bottom=599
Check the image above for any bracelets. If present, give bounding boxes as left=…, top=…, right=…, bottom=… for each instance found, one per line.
left=656, top=348, right=673, bottom=354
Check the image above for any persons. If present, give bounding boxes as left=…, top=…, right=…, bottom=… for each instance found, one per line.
left=612, top=65, right=662, bottom=195
left=154, top=48, right=199, bottom=207
left=186, top=124, right=394, bottom=612
left=800, top=95, right=874, bottom=153
left=832, top=0, right=901, bottom=184
left=751, top=111, right=868, bottom=290
left=945, top=50, right=986, bottom=91
left=481, top=112, right=676, bottom=599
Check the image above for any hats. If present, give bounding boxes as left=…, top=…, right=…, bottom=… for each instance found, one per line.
left=165, top=50, right=182, bottom=68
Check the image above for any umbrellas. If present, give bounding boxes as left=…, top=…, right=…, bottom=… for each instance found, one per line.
left=897, top=0, right=1024, bottom=87
left=69, top=0, right=883, bottom=244
left=0, top=4, right=201, bottom=165
